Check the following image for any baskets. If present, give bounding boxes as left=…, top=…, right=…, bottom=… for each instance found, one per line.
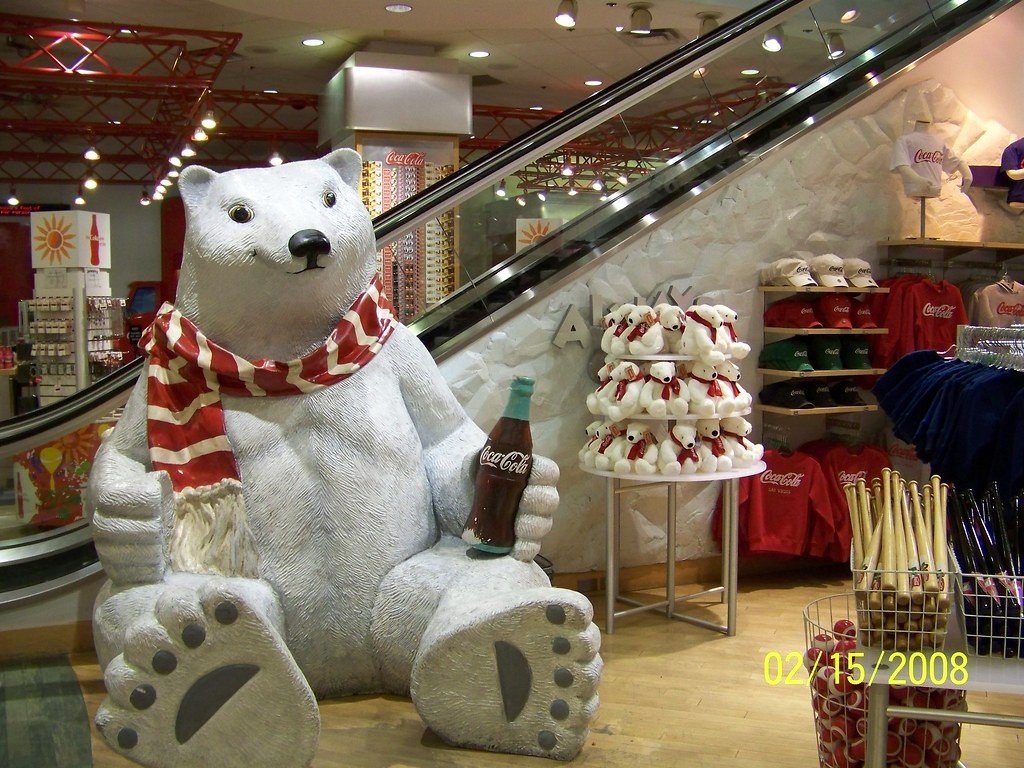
left=803, top=589, right=1024, bottom=768
left=848, top=537, right=1024, bottom=660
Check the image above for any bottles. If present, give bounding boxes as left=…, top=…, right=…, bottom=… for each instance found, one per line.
left=460, top=375, right=536, bottom=557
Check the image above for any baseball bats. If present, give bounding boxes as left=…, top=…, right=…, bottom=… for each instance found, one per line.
left=843, top=467, right=1023, bottom=660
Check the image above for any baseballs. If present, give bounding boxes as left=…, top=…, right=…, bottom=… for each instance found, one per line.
left=803, top=619, right=968, bottom=767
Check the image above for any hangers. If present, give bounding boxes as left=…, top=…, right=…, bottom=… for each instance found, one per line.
left=825, top=420, right=865, bottom=449
left=937, top=326, right=1024, bottom=374
left=764, top=422, right=793, bottom=455
left=890, top=255, right=939, bottom=282
left=967, top=260, right=1015, bottom=285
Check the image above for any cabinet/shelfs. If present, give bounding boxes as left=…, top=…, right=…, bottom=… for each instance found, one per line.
left=755, top=284, right=891, bottom=417
left=16, top=212, right=131, bottom=405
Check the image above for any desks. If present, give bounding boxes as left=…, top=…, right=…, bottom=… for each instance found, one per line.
left=854, top=603, right=1024, bottom=768
left=0, top=365, right=19, bottom=421
left=577, top=459, right=767, bottom=637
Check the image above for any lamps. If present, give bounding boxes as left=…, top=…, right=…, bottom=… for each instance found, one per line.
left=560, top=156, right=576, bottom=176
left=617, top=168, right=630, bottom=186
left=201, top=111, right=217, bottom=129
left=835, top=0, right=861, bottom=24
left=4, top=146, right=183, bottom=207
left=535, top=187, right=550, bottom=202
left=496, top=179, right=507, bottom=197
left=554, top=0, right=579, bottom=28
left=193, top=128, right=207, bottom=142
left=592, top=171, right=605, bottom=191
left=269, top=152, right=283, bottom=166
left=567, top=177, right=578, bottom=197
left=823, top=29, right=847, bottom=60
left=761, top=24, right=786, bottom=54
left=600, top=187, right=608, bottom=202
left=516, top=189, right=528, bottom=205
left=696, top=11, right=723, bottom=38
left=627, top=1, right=654, bottom=34
left=181, top=144, right=196, bottom=156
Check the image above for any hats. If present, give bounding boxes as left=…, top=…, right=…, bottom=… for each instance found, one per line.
left=841, top=335, right=871, bottom=369
left=808, top=253, right=849, bottom=287
left=807, top=335, right=843, bottom=370
left=758, top=340, right=815, bottom=371
left=759, top=258, right=819, bottom=287
left=817, top=293, right=853, bottom=329
left=763, top=297, right=823, bottom=328
left=842, top=257, right=879, bottom=288
left=804, top=379, right=839, bottom=407
left=853, top=299, right=878, bottom=329
left=826, top=379, right=866, bottom=406
left=11, top=342, right=33, bottom=354
left=758, top=381, right=815, bottom=409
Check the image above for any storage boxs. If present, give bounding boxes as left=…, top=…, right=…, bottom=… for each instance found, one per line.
left=849, top=534, right=957, bottom=653
left=949, top=541, right=1024, bottom=663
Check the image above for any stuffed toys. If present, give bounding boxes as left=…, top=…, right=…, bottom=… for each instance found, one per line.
left=577, top=303, right=764, bottom=475
